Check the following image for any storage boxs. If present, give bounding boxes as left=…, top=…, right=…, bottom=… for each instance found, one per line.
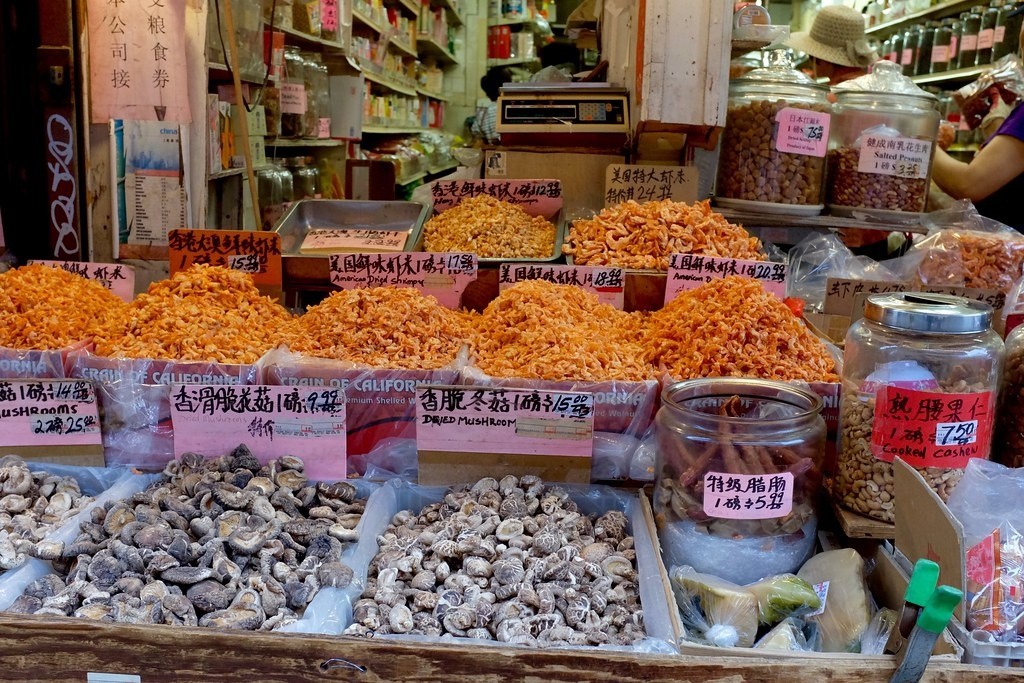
left=0, top=200, right=1024, bottom=683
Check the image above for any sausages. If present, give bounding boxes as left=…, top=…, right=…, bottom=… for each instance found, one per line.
left=669, top=395, right=820, bottom=527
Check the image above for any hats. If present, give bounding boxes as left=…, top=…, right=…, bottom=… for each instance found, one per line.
left=780, top=3, right=880, bottom=69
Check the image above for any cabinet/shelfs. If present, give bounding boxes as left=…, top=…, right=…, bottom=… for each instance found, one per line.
left=864, top=0, right=1023, bottom=153
left=205, top=0, right=559, bottom=230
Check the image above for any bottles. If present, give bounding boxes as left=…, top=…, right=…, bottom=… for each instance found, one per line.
left=861, top=0, right=1024, bottom=77
left=716, top=46, right=833, bottom=225
left=258, top=44, right=333, bottom=230
left=651, top=377, right=827, bottom=586
left=822, top=59, right=944, bottom=221
left=990, top=323, right=1024, bottom=469
left=836, top=292, right=1006, bottom=525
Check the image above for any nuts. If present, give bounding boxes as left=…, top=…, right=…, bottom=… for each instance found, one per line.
left=837, top=366, right=999, bottom=523
left=832, top=134, right=926, bottom=214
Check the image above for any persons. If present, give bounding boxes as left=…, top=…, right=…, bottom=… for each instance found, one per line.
left=932, top=12, right=1024, bottom=236
left=779, top=4, right=877, bottom=85
left=472, top=66, right=512, bottom=143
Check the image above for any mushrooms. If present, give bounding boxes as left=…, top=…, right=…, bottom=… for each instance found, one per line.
left=0, top=442, right=647, bottom=652
